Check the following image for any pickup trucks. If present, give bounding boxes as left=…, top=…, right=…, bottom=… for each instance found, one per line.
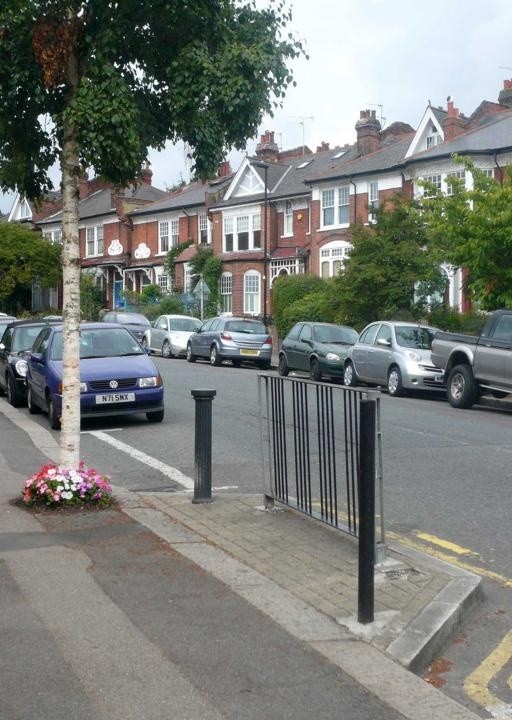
left=430, top=310, right=511, bottom=407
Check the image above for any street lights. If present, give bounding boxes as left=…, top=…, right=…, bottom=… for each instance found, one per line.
left=249, top=160, right=270, bottom=324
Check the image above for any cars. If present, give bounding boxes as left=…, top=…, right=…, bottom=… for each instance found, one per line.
left=103, top=312, right=153, bottom=348
left=26, top=325, right=166, bottom=431
left=186, top=317, right=273, bottom=370
left=344, top=320, right=449, bottom=396
left=0, top=319, right=62, bottom=407
left=142, top=314, right=205, bottom=357
left=278, top=322, right=360, bottom=380
